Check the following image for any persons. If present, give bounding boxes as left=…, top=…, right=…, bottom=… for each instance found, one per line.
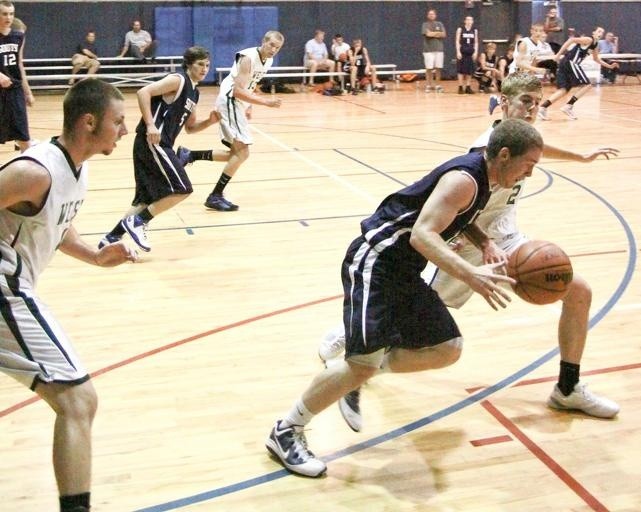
left=318, top=66, right=624, bottom=433
left=117, top=19, right=160, bottom=64
left=419, top=2, right=620, bottom=121
left=0, top=1, right=37, bottom=152
left=97, top=46, right=224, bottom=251
left=176, top=31, right=286, bottom=212
left=68, top=30, right=101, bottom=84
left=264, top=115, right=546, bottom=479
left=303, top=27, right=388, bottom=95
left=1, top=77, right=142, bottom=512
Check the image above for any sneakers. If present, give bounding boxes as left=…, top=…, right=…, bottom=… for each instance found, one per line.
left=425, top=86, right=473, bottom=94
left=317, top=335, right=346, bottom=361
left=99, top=235, right=139, bottom=262
left=537, top=106, right=551, bottom=120
left=121, top=214, right=152, bottom=252
left=488, top=95, right=497, bottom=114
left=205, top=194, right=239, bottom=211
left=547, top=382, right=619, bottom=418
left=339, top=387, right=362, bottom=433
left=177, top=146, right=192, bottom=166
left=560, top=104, right=577, bottom=120
left=264, top=419, right=327, bottom=478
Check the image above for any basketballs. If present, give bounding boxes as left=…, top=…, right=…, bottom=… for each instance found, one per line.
left=507, top=242, right=571, bottom=301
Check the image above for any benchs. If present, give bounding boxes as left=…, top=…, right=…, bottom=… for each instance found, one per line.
left=221, top=69, right=434, bottom=93
left=215, top=63, right=399, bottom=73
left=11, top=54, right=185, bottom=93
